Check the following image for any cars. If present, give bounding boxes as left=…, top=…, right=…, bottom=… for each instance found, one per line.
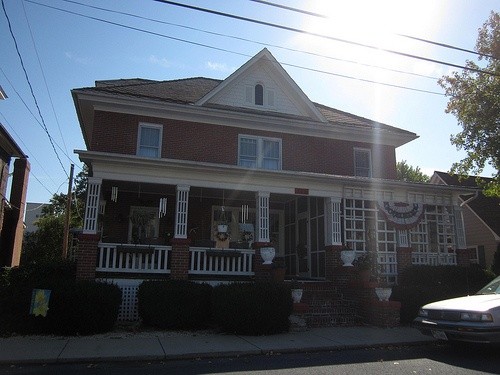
left=414, top=276, right=500, bottom=352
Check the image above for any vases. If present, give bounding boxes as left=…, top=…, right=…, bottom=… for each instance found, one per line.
left=260, top=246, right=275, bottom=264
left=217, top=224, right=228, bottom=233
left=340, top=251, right=355, bottom=267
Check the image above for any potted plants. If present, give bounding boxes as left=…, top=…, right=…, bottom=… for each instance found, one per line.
left=351, top=251, right=378, bottom=289
left=374, top=281, right=392, bottom=302
left=290, top=280, right=303, bottom=304
left=273, top=256, right=287, bottom=286
